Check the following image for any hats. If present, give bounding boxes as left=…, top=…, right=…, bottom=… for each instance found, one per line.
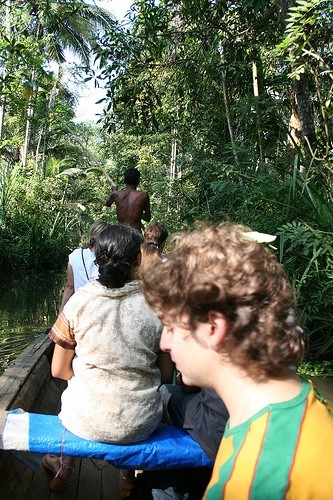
left=244, top=231, right=277, bottom=243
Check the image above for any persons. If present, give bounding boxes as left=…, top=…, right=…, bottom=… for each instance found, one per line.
left=161, top=370, right=228, bottom=459
left=139, top=221, right=333, bottom=500
left=139, top=222, right=168, bottom=277
left=40, top=225, right=162, bottom=497
left=106, top=168, right=151, bottom=232
left=60, top=222, right=110, bottom=315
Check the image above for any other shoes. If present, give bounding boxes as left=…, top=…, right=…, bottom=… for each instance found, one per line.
left=152, top=487, right=188, bottom=500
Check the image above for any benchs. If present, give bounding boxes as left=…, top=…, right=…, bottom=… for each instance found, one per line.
left=0, top=407, right=213, bottom=498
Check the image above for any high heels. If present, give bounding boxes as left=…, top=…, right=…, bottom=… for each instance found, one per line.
left=42, top=453, right=74, bottom=491
left=118, top=468, right=135, bottom=500
